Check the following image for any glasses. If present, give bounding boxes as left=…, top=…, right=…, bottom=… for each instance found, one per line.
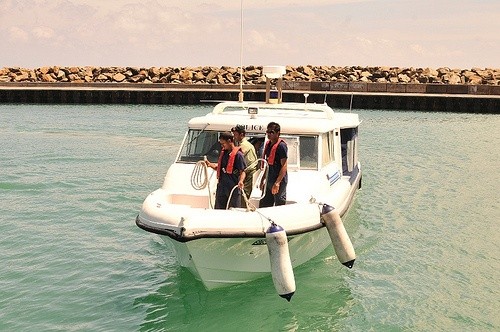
left=266, top=130, right=276, bottom=135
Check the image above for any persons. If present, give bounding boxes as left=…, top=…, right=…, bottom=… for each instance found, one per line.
left=200, top=132, right=247, bottom=209
left=259, top=122, right=288, bottom=209
left=231, top=125, right=258, bottom=208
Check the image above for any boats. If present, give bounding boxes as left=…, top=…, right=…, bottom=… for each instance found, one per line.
left=135, top=1, right=363, bottom=292
